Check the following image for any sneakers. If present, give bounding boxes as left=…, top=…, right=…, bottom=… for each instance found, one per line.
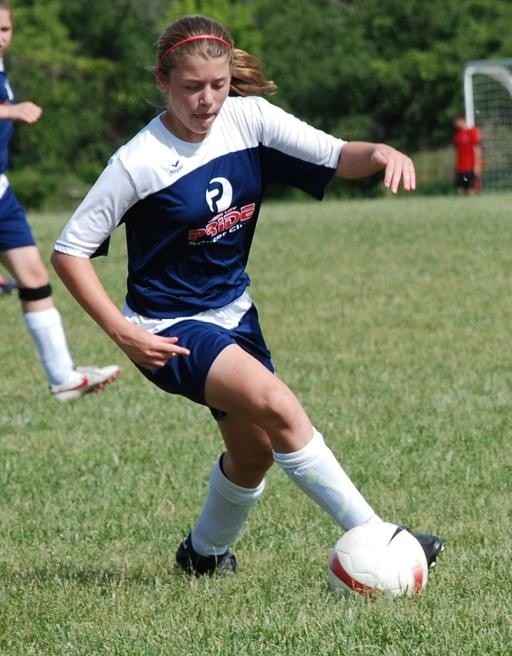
left=413, top=533, right=445, bottom=573
left=50, top=365, right=121, bottom=403
left=176, top=531, right=240, bottom=578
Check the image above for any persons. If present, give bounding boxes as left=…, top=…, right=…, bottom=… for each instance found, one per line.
left=49, top=16, right=444, bottom=581
left=448, top=115, right=483, bottom=197
left=0, top=0, right=121, bottom=403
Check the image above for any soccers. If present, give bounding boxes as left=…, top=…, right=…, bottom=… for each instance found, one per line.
left=329, top=522, right=428, bottom=597
